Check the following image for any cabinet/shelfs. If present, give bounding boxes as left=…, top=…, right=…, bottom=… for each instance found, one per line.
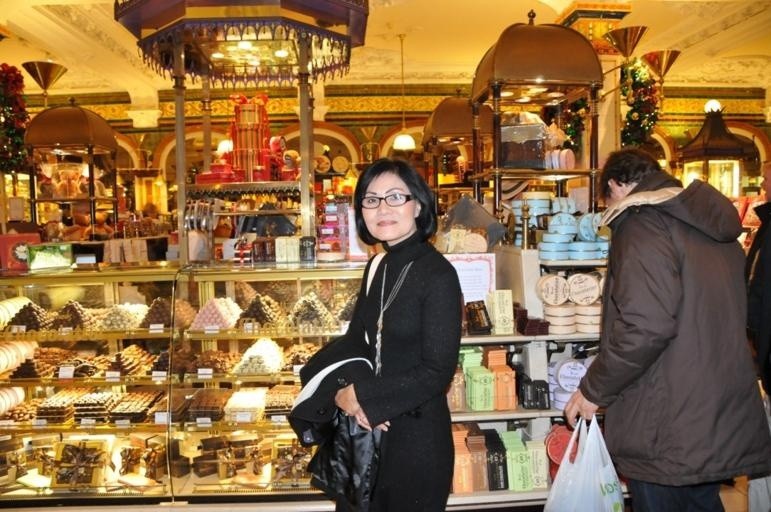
left=0, top=261, right=175, bottom=507
left=449, top=237, right=608, bottom=511
left=178, top=262, right=363, bottom=511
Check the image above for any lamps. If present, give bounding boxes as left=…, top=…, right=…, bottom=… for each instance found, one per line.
left=390, top=31, right=416, bottom=152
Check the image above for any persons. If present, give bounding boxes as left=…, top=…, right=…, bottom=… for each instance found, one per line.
left=285, top=158, right=463, bottom=512
left=564, top=147, right=771, bottom=511
left=743, top=159, right=771, bottom=396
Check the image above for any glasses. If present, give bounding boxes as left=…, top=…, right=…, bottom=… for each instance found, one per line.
left=362, top=194, right=417, bottom=209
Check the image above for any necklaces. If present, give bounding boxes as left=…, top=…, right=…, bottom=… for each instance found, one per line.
left=372, top=259, right=413, bottom=376
